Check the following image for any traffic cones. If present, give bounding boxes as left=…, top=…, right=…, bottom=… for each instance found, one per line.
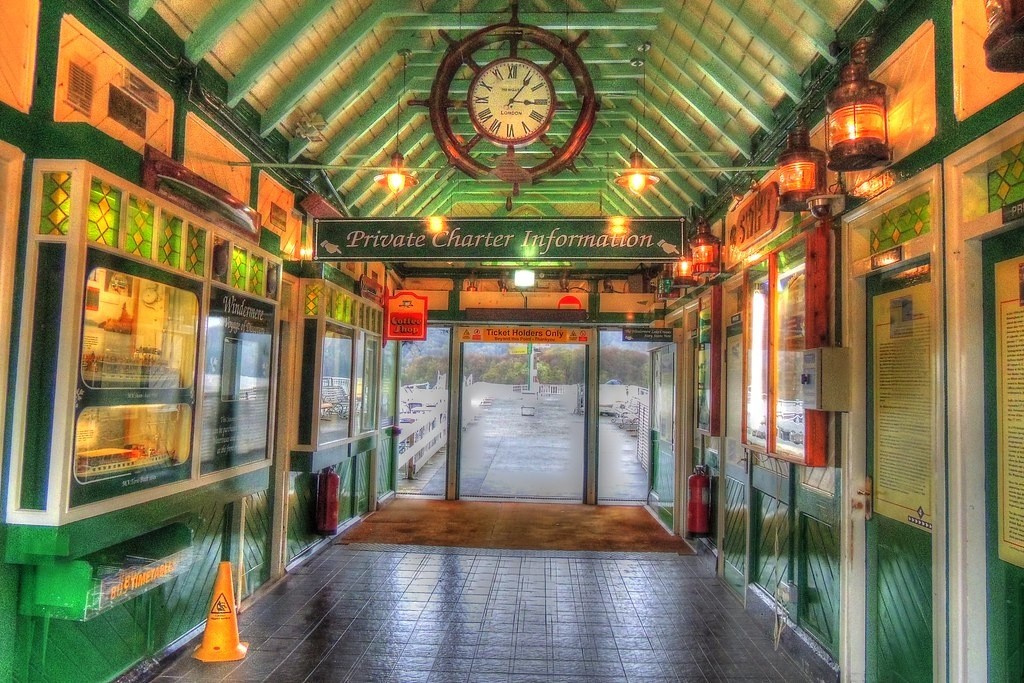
left=191, top=561, right=249, bottom=663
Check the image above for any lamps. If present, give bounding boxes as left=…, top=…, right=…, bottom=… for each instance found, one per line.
left=373, top=94, right=419, bottom=214
left=827, top=58, right=893, bottom=171
left=612, top=59, right=660, bottom=196
left=777, top=113, right=826, bottom=213
left=983, top=0, right=1024, bottom=72
left=687, top=216, right=720, bottom=278
left=672, top=255, right=698, bottom=288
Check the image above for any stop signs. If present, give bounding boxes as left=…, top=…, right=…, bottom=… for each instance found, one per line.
left=557, top=295, right=582, bottom=311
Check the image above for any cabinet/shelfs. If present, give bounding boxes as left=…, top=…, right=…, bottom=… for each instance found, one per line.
left=0, top=160, right=385, bottom=565
left=697, top=283, right=721, bottom=436
left=739, top=226, right=829, bottom=466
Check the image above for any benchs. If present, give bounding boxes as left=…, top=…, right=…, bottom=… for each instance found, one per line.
left=321, top=385, right=362, bottom=420
left=610, top=397, right=640, bottom=429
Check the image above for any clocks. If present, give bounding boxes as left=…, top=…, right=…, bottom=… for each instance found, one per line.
left=408, top=5, right=609, bottom=209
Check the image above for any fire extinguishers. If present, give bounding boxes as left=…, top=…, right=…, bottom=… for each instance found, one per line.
left=686, top=465, right=712, bottom=539
left=316, top=465, right=340, bottom=536
